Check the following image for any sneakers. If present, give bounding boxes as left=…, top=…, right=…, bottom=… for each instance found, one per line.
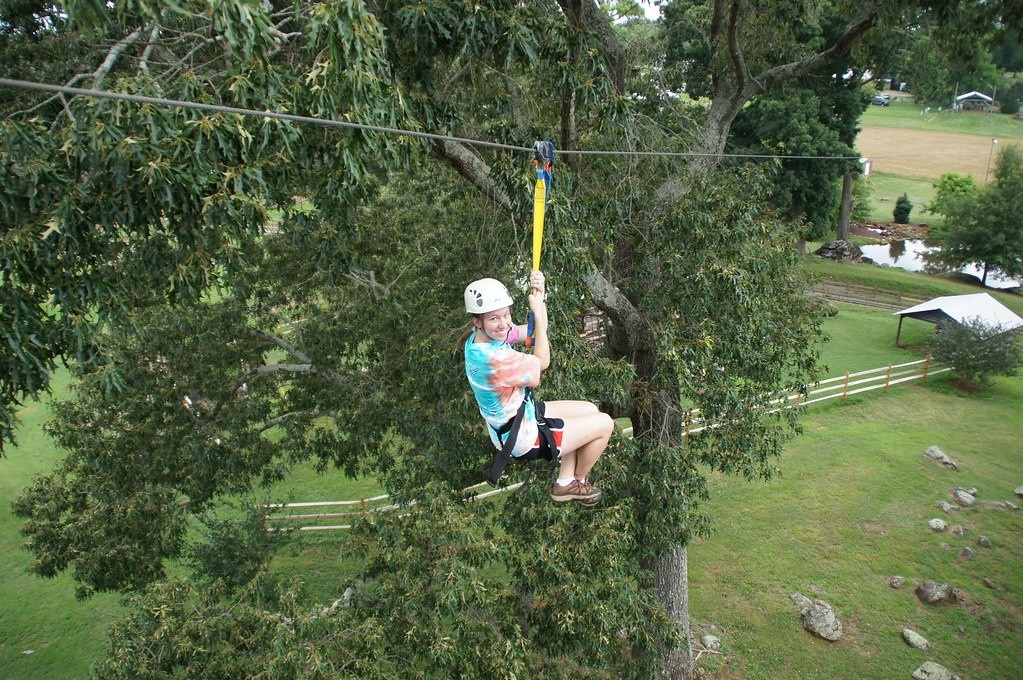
left=571, top=475, right=601, bottom=506
left=550, top=480, right=602, bottom=504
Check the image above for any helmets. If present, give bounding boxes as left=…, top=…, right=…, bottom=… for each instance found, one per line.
left=464, top=278, right=514, bottom=314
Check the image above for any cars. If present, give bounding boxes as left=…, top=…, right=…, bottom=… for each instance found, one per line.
left=872, top=96, right=889, bottom=106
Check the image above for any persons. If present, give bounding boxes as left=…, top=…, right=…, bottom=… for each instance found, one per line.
left=464, top=271, right=614, bottom=506
